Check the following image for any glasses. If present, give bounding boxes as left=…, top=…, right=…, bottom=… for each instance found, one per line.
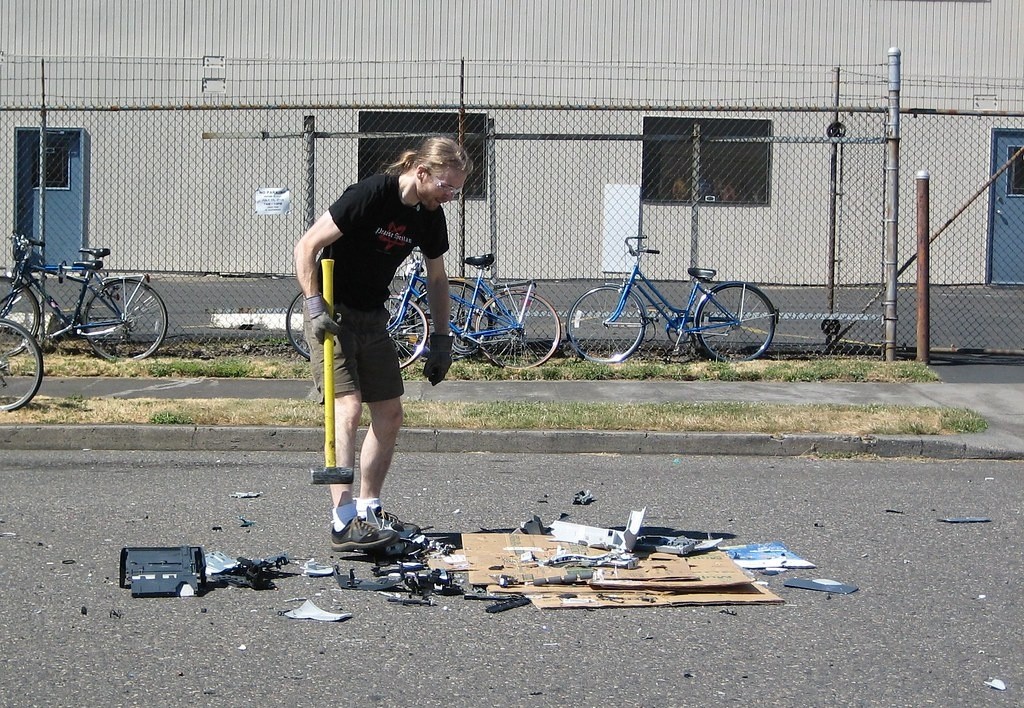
left=428, top=171, right=461, bottom=197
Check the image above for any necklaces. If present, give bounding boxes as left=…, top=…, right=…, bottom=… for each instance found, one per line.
left=402, top=197, right=423, bottom=211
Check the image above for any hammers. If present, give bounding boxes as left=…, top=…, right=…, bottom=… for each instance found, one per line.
left=309, top=258, right=355, bottom=486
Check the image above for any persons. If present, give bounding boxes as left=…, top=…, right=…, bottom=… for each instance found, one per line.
left=294, top=136, right=473, bottom=552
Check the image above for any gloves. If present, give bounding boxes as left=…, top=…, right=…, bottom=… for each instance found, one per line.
left=423, top=332, right=455, bottom=387
left=307, top=292, right=341, bottom=346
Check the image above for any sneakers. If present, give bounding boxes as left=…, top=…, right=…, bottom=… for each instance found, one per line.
left=358, top=505, right=420, bottom=539
left=331, top=516, right=398, bottom=552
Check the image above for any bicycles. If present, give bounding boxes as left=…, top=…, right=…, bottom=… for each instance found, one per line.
left=562, top=234, right=779, bottom=364
left=285, top=245, right=562, bottom=371
left=0, top=225, right=169, bottom=415
